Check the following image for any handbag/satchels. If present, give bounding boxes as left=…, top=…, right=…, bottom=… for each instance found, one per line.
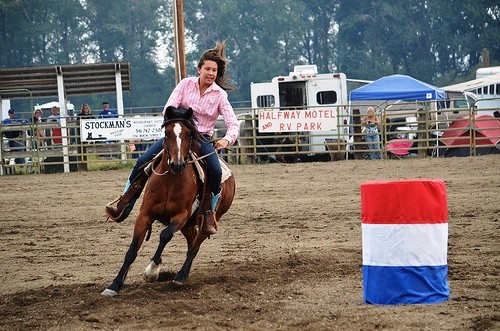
left=366, top=125, right=378, bottom=137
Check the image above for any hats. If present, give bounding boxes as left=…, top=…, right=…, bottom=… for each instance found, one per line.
left=8, top=108, right=15, bottom=115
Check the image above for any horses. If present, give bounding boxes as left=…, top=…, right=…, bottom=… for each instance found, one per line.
left=101, top=106, right=236, bottom=296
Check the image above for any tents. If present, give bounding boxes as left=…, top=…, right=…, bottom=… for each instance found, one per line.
left=433, top=113, right=500, bottom=158
left=344, top=74, right=445, bottom=159
left=34, top=101, right=74, bottom=110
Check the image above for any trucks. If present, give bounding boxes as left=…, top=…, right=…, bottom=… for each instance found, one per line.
left=250, top=65, right=354, bottom=158
left=35, top=100, right=78, bottom=146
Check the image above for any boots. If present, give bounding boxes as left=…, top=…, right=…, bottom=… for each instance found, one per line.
left=205, top=211, right=217, bottom=235
left=106, top=205, right=126, bottom=223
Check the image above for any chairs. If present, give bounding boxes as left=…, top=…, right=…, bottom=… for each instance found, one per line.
left=388, top=139, right=414, bottom=159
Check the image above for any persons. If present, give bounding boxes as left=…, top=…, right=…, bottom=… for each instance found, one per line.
left=46, top=106, right=71, bottom=150
left=76, top=103, right=96, bottom=124
left=27, top=108, right=49, bottom=149
left=105, top=42, right=240, bottom=236
left=97, top=102, right=118, bottom=144
left=1, top=109, right=29, bottom=165
left=360, top=106, right=383, bottom=159
